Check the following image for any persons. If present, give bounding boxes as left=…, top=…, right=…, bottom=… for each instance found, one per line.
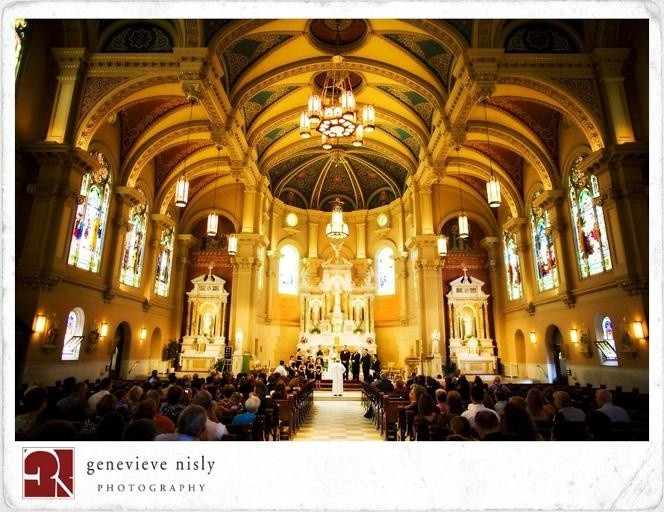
left=15, top=369, right=313, bottom=441
left=364, top=372, right=632, bottom=441
left=274, top=345, right=380, bottom=396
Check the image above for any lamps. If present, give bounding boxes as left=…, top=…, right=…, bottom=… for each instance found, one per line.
left=173, top=102, right=241, bottom=255
left=324, top=139, right=349, bottom=240
left=298, top=54, right=376, bottom=151
left=436, top=101, right=502, bottom=259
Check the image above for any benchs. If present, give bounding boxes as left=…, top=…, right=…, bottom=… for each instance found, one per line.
left=272, top=382, right=313, bottom=440
left=361, top=378, right=412, bottom=440
